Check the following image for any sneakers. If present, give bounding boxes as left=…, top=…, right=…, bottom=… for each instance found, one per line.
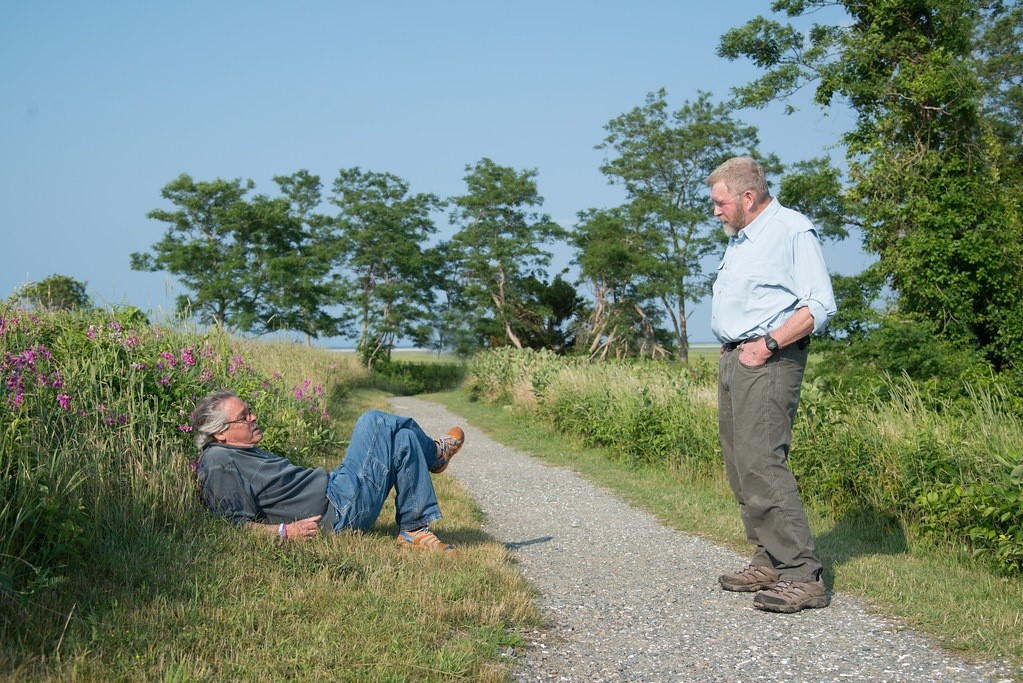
left=427, top=426, right=464, bottom=473
left=397, top=527, right=456, bottom=553
left=752, top=575, right=828, bottom=613
left=718, top=565, right=777, bottom=592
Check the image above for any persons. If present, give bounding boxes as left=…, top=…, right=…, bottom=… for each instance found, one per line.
left=705, top=155, right=837, bottom=612
left=190, top=391, right=465, bottom=552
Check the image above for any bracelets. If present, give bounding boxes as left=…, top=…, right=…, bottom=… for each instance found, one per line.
left=279, top=524, right=287, bottom=540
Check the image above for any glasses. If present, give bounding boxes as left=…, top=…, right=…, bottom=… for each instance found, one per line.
left=223, top=407, right=252, bottom=424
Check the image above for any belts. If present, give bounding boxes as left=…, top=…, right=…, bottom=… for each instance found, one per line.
left=726, top=339, right=747, bottom=353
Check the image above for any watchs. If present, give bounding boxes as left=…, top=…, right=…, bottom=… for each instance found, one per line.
left=765, top=335, right=779, bottom=353
